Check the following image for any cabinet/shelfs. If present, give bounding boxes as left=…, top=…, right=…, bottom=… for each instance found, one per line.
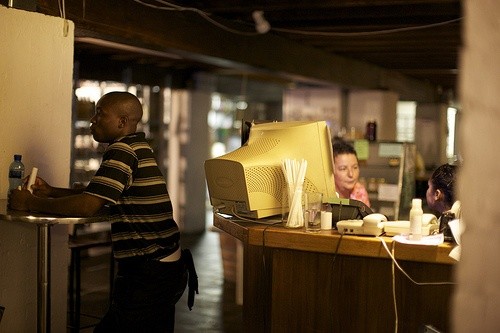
left=360, top=140, right=418, bottom=217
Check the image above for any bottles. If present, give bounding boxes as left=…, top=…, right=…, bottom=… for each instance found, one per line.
left=409, top=198, right=423, bottom=237
left=8, top=154, right=24, bottom=204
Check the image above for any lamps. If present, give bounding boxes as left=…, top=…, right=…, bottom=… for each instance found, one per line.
left=254, top=11, right=270, bottom=33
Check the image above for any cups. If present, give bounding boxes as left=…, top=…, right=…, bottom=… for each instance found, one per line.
left=305, top=193, right=321, bottom=230
left=282, top=187, right=305, bottom=229
left=321, top=210, right=332, bottom=230
left=439, top=212, right=455, bottom=241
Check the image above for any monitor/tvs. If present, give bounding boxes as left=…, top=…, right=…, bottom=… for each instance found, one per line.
left=204, top=123, right=338, bottom=218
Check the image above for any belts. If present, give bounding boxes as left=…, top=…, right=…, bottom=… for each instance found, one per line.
left=157, top=245, right=182, bottom=263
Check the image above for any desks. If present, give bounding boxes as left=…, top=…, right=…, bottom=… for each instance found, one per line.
left=211, top=211, right=465, bottom=333
left=0, top=197, right=119, bottom=333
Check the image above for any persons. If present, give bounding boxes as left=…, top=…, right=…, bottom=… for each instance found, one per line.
left=8, top=92, right=198, bottom=333
left=331, top=136, right=370, bottom=209
left=426, top=163, right=462, bottom=235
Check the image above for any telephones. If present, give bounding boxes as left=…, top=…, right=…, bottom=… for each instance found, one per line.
left=336, top=213, right=387, bottom=237
left=380, top=214, right=440, bottom=237
